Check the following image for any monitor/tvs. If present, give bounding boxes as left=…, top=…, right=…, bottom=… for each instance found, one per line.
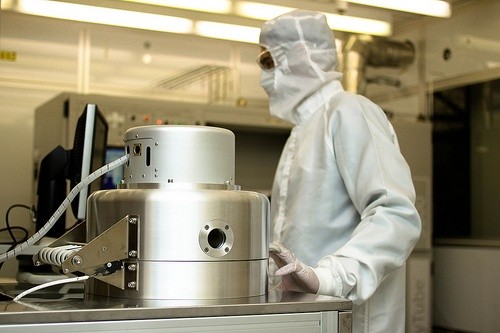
left=102, top=146, right=129, bottom=189
left=36, top=103, right=108, bottom=248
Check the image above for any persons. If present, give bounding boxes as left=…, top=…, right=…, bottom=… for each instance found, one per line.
left=256, top=9, right=422, bottom=332
